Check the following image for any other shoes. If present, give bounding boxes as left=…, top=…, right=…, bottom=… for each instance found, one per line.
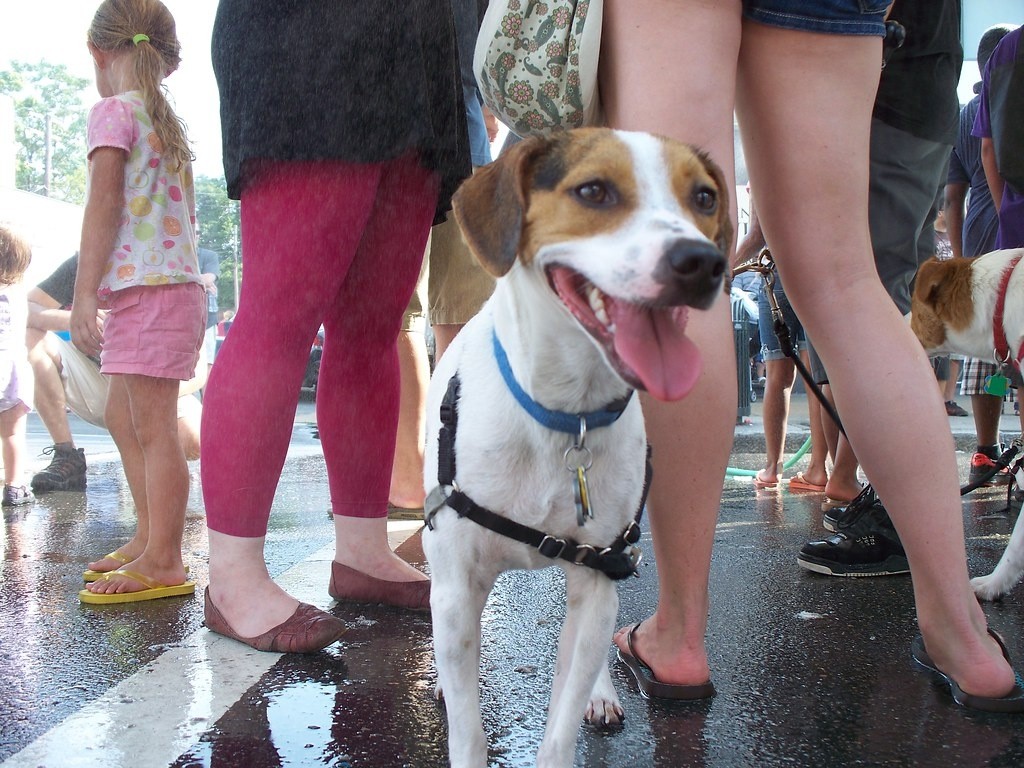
left=204, top=581, right=348, bottom=653
left=328, top=561, right=432, bottom=611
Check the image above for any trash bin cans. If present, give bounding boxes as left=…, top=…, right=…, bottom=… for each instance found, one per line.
left=731, top=285, right=753, bottom=427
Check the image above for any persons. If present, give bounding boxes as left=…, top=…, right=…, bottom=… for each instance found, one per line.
left=0, top=227, right=218, bottom=504
left=199, top=0, right=1024, bottom=710
left=71, top=0, right=207, bottom=603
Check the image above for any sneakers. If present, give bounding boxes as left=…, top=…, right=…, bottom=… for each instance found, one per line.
left=2, top=482, right=34, bottom=506
left=945, top=400, right=968, bottom=416
left=822, top=483, right=881, bottom=534
left=31, top=441, right=88, bottom=492
left=968, top=452, right=1013, bottom=487
left=797, top=499, right=913, bottom=576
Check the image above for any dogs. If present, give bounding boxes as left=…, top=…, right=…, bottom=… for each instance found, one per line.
left=907, top=249, right=1023, bottom=603
left=419, top=124, right=736, bottom=767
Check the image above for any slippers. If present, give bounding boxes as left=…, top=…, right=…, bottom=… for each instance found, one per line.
left=79, top=568, right=198, bottom=604
left=328, top=495, right=425, bottom=520
left=84, top=549, right=190, bottom=580
left=913, top=627, right=1024, bottom=711
left=618, top=619, right=715, bottom=700
left=789, top=472, right=826, bottom=491
left=753, top=468, right=777, bottom=488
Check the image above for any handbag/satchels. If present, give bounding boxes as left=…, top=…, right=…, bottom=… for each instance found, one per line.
left=473, top=0, right=605, bottom=143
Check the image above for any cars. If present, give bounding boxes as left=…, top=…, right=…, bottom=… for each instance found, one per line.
left=212, top=303, right=434, bottom=395
